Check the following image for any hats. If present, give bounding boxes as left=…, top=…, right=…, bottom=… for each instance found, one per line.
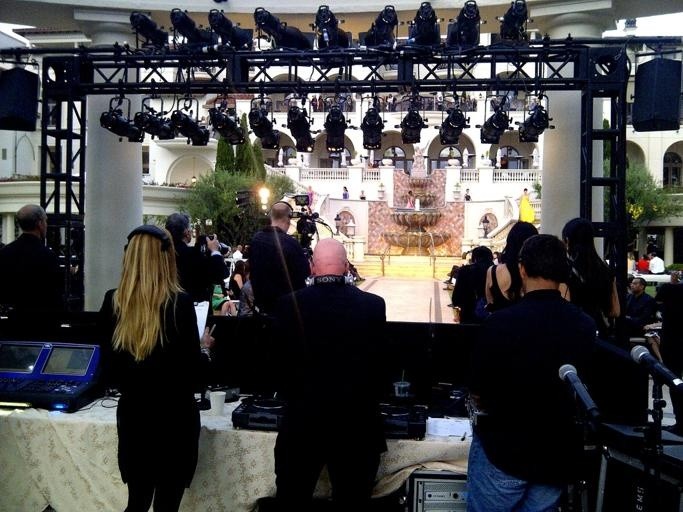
left=166, top=212, right=191, bottom=235
left=520, top=234, right=577, bottom=282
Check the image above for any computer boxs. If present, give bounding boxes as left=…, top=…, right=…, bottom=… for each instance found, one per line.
left=405, top=471, right=469, bottom=512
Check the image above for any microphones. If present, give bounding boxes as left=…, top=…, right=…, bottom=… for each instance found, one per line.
left=558, top=364, right=603, bottom=419
left=630, top=345, right=683, bottom=394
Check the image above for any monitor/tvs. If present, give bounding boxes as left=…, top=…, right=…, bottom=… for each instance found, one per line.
left=0, top=342, right=43, bottom=374
left=41, top=345, right=96, bottom=376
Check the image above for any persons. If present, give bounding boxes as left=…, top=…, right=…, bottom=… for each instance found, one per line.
left=306, top=89, right=541, bottom=111
left=372, top=159, right=378, bottom=168
left=167, top=173, right=385, bottom=336
left=465, top=235, right=596, bottom=512
left=656, top=271, right=682, bottom=438
left=266, top=242, right=386, bottom=511
left=501, top=154, right=508, bottom=168
left=0, top=204, right=65, bottom=340
left=443, top=186, right=682, bottom=343
left=100, top=225, right=214, bottom=512
left=407, top=190, right=415, bottom=208
left=368, top=162, right=372, bottom=168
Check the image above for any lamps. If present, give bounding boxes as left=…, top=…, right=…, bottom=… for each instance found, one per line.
left=134, top=94, right=178, bottom=140
left=254, top=7, right=315, bottom=67
left=394, top=92, right=428, bottom=144
left=360, top=95, right=387, bottom=150
left=287, top=97, right=317, bottom=153
left=171, top=93, right=210, bottom=146
left=309, top=5, right=352, bottom=70
left=323, top=93, right=351, bottom=154
left=248, top=94, right=281, bottom=149
left=407, top=2, right=440, bottom=45
left=100, top=94, right=145, bottom=143
left=208, top=9, right=254, bottom=50
left=434, top=91, right=470, bottom=145
left=491, top=0, right=528, bottom=44
left=129, top=11, right=168, bottom=48
left=475, top=91, right=514, bottom=144
left=208, top=94, right=245, bottom=145
left=515, top=91, right=555, bottom=143
left=358, top=5, right=399, bottom=67
left=170, top=8, right=219, bottom=48
left=447, top=0, right=481, bottom=46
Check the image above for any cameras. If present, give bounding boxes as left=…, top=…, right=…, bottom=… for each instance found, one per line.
left=193, top=234, right=230, bottom=258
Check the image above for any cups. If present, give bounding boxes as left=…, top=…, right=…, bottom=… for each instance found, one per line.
left=394, top=381, right=410, bottom=398
left=210, top=391, right=226, bottom=417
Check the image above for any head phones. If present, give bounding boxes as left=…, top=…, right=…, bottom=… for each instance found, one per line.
left=273, top=201, right=294, bottom=218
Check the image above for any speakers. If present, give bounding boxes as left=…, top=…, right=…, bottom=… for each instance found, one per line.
left=632, top=57, right=681, bottom=132
left=0, top=67, right=41, bottom=132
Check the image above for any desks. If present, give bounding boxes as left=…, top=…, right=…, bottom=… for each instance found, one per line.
left=0, top=395, right=474, bottom=512
